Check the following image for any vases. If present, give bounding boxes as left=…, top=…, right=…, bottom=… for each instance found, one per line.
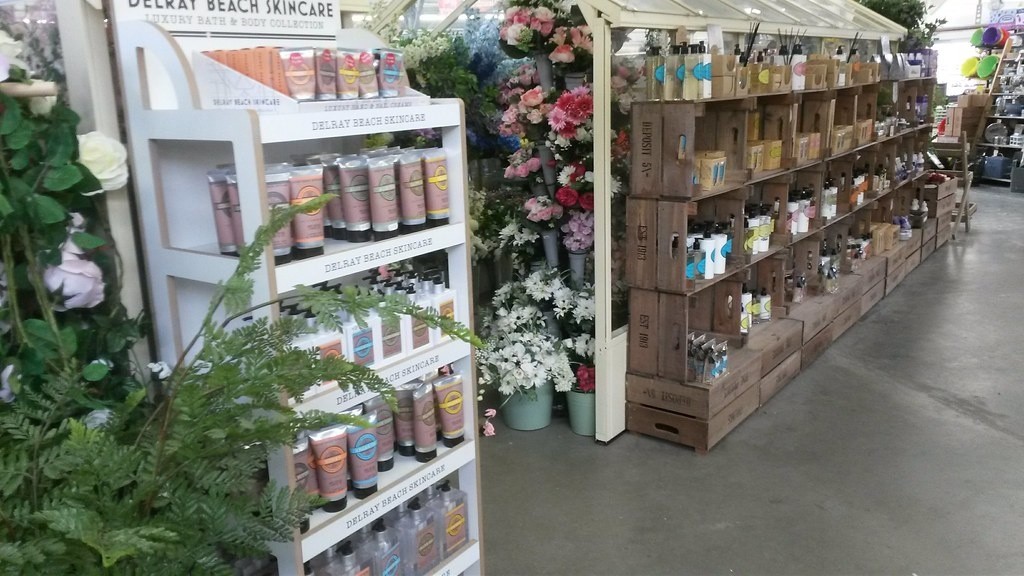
left=498, top=375, right=553, bottom=430
left=564, top=390, right=595, bottom=436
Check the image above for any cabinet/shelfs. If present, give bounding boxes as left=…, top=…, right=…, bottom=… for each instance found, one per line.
left=931, top=130, right=979, bottom=233
left=969, top=38, right=1024, bottom=182
left=117, top=21, right=481, bottom=576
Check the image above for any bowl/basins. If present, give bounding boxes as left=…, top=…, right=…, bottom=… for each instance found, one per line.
left=961, top=55, right=1001, bottom=80
left=970, top=26, right=1011, bottom=49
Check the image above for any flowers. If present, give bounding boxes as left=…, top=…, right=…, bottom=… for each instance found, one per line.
left=358, top=0, right=641, bottom=402
left=0, top=31, right=171, bottom=440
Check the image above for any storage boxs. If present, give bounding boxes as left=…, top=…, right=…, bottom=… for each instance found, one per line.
left=984, top=156, right=1011, bottom=178
left=1010, top=168, right=1024, bottom=191
left=943, top=92, right=991, bottom=137
left=625, top=76, right=958, bottom=454
